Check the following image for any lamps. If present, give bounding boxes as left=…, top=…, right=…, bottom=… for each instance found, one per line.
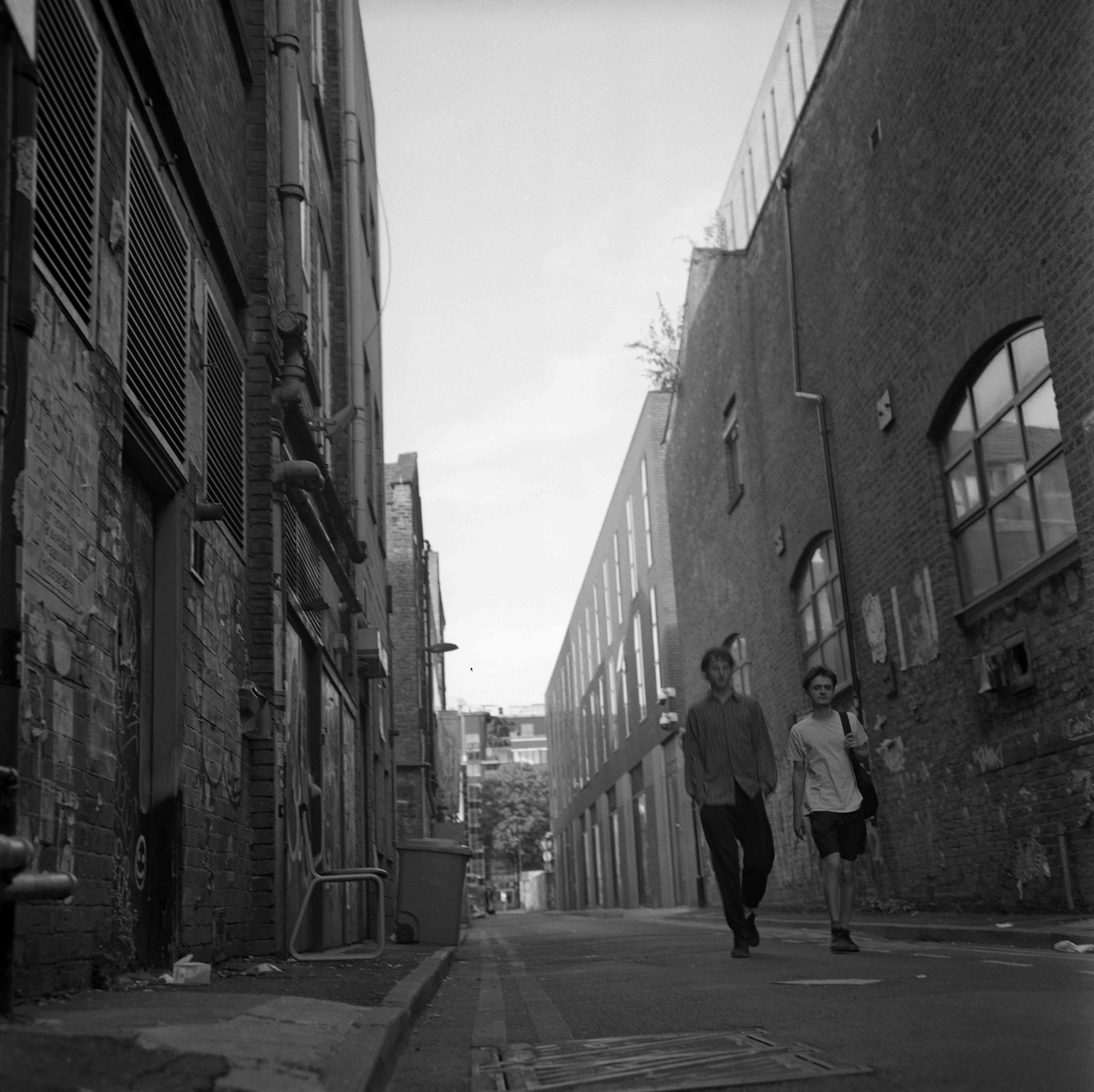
left=421, top=641, right=459, bottom=653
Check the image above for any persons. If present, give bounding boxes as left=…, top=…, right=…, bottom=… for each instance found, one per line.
left=684, top=646, right=778, bottom=959
left=786, top=666, right=872, bottom=954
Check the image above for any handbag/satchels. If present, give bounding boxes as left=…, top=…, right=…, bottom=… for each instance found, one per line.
left=838, top=710, right=878, bottom=820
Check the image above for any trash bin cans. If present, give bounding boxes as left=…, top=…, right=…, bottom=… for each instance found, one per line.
left=391, top=836, right=473, bottom=947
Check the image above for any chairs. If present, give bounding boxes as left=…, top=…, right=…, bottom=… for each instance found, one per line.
left=289, top=803, right=388, bottom=961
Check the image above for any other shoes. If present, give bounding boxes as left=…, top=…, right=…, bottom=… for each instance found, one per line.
left=829, top=928, right=859, bottom=953
left=742, top=910, right=760, bottom=947
left=731, top=938, right=750, bottom=958
